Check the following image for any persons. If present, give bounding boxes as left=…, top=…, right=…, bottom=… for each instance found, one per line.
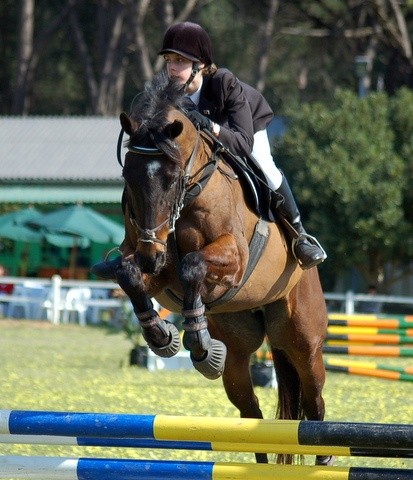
left=93, top=21, right=326, bottom=277
left=0, top=249, right=31, bottom=316
left=362, top=285, right=381, bottom=315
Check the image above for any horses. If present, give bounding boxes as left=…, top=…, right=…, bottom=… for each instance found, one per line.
left=86, top=57, right=334, bottom=468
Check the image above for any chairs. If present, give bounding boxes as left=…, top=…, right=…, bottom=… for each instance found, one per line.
left=7, top=286, right=111, bottom=324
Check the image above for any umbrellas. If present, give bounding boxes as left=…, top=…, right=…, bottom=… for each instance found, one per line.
left=0, top=203, right=126, bottom=279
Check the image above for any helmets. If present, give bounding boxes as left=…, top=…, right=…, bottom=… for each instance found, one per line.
left=158, top=22, right=212, bottom=65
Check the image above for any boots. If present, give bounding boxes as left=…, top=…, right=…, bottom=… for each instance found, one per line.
left=269, top=168, right=323, bottom=266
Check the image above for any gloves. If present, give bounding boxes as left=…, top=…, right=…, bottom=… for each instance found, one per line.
left=185, top=109, right=213, bottom=133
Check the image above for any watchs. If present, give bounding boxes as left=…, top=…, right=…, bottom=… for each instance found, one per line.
left=211, top=122, right=220, bottom=136
